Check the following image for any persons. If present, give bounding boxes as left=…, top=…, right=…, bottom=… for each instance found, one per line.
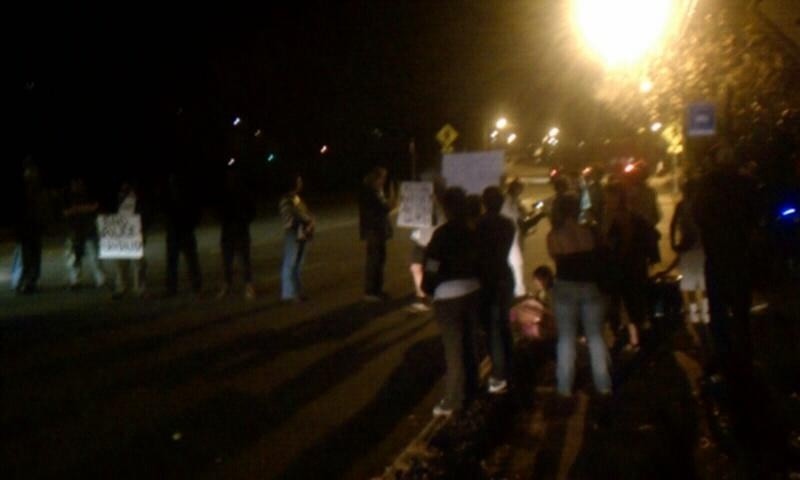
left=62, top=172, right=107, bottom=289
left=210, top=172, right=257, bottom=300
left=11, top=163, right=54, bottom=297
left=358, top=163, right=399, bottom=304
left=407, top=112, right=800, bottom=416
left=275, top=172, right=318, bottom=305
left=159, top=169, right=204, bottom=297
left=100, top=182, right=150, bottom=300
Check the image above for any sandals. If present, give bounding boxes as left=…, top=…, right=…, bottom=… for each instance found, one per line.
left=614, top=340, right=641, bottom=357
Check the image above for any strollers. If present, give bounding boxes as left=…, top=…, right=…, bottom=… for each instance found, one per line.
left=510, top=291, right=560, bottom=347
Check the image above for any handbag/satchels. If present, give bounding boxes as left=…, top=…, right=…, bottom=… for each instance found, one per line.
left=298, top=222, right=314, bottom=241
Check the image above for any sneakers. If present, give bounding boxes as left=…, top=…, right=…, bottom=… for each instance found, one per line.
left=555, top=385, right=573, bottom=397
left=597, top=386, right=611, bottom=395
left=432, top=401, right=452, bottom=416
left=486, top=377, right=508, bottom=393
